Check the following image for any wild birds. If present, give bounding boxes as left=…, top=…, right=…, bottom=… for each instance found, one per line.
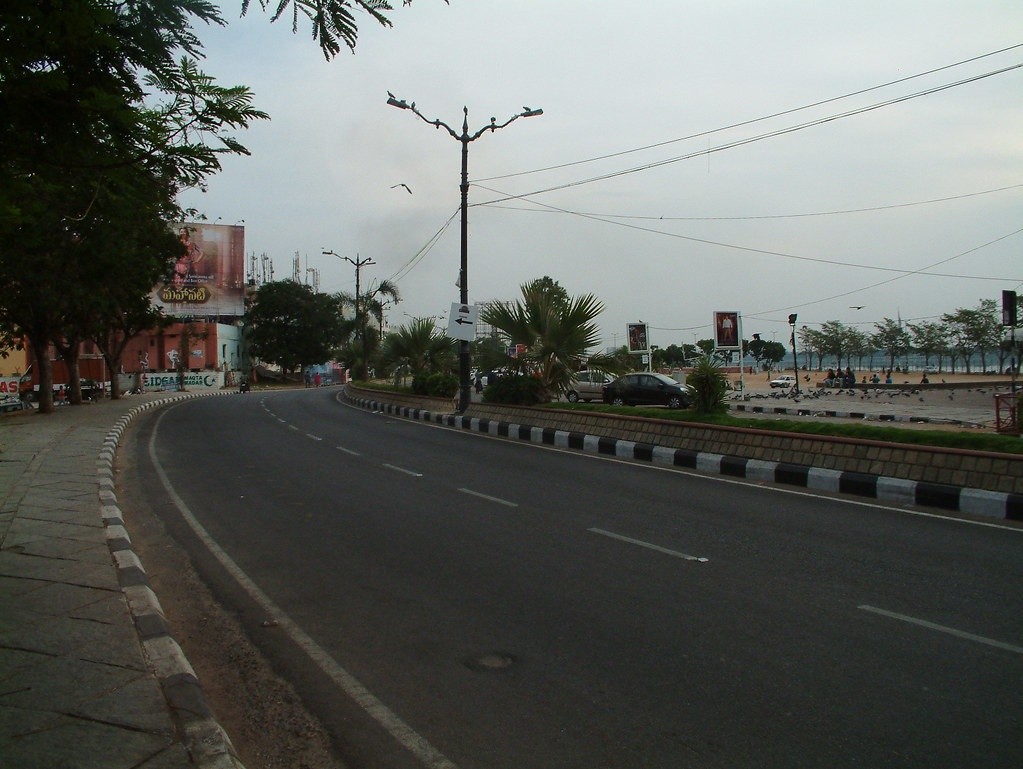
left=387, top=90, right=396, bottom=100
left=728, top=374, right=1012, bottom=404
left=850, top=305, right=868, bottom=310
left=390, top=184, right=413, bottom=194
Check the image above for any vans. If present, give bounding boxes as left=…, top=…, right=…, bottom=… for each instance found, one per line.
left=771, top=375, right=796, bottom=388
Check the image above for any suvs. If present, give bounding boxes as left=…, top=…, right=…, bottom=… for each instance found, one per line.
left=564, top=370, right=617, bottom=403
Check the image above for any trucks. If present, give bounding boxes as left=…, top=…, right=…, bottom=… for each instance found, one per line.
left=19, top=354, right=111, bottom=402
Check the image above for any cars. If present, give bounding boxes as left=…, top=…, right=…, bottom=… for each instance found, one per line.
left=470, top=365, right=523, bottom=389
left=602, top=373, right=698, bottom=409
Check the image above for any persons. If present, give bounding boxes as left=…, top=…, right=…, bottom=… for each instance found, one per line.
left=868, top=373, right=880, bottom=384
left=722, top=315, right=734, bottom=345
left=920, top=374, right=929, bottom=384
left=822, top=369, right=835, bottom=388
left=843, top=367, right=856, bottom=388
left=885, top=374, right=893, bottom=383
left=831, top=368, right=845, bottom=388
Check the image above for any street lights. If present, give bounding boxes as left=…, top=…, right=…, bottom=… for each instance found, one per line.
left=387, top=97, right=544, bottom=415
left=403, top=313, right=436, bottom=331
left=788, top=313, right=799, bottom=395
left=322, top=252, right=377, bottom=341
